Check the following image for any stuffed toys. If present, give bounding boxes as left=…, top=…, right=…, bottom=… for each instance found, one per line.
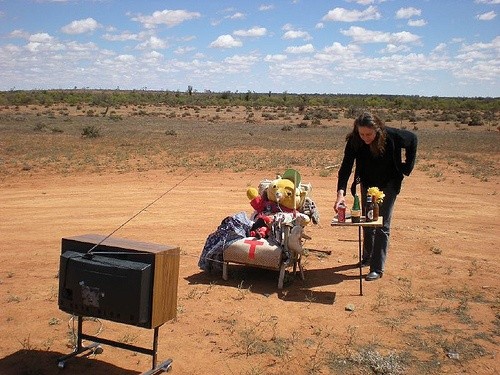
left=247, top=173, right=308, bottom=239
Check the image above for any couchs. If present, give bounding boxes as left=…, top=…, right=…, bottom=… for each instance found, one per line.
left=222, top=181, right=311, bottom=290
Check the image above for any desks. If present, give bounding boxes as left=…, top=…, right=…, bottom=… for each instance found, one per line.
left=330, top=213, right=384, bottom=295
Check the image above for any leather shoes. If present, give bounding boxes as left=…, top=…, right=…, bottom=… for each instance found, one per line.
left=365, top=271, right=382, bottom=281
left=358, top=259, right=370, bottom=266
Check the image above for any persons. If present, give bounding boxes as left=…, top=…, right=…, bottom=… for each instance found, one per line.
left=333, top=112, right=418, bottom=281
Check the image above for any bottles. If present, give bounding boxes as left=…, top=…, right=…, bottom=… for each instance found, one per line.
left=365, top=195, right=373, bottom=222
left=351, top=194, right=361, bottom=223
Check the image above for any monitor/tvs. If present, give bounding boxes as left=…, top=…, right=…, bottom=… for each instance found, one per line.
left=58, top=171, right=196, bottom=329
